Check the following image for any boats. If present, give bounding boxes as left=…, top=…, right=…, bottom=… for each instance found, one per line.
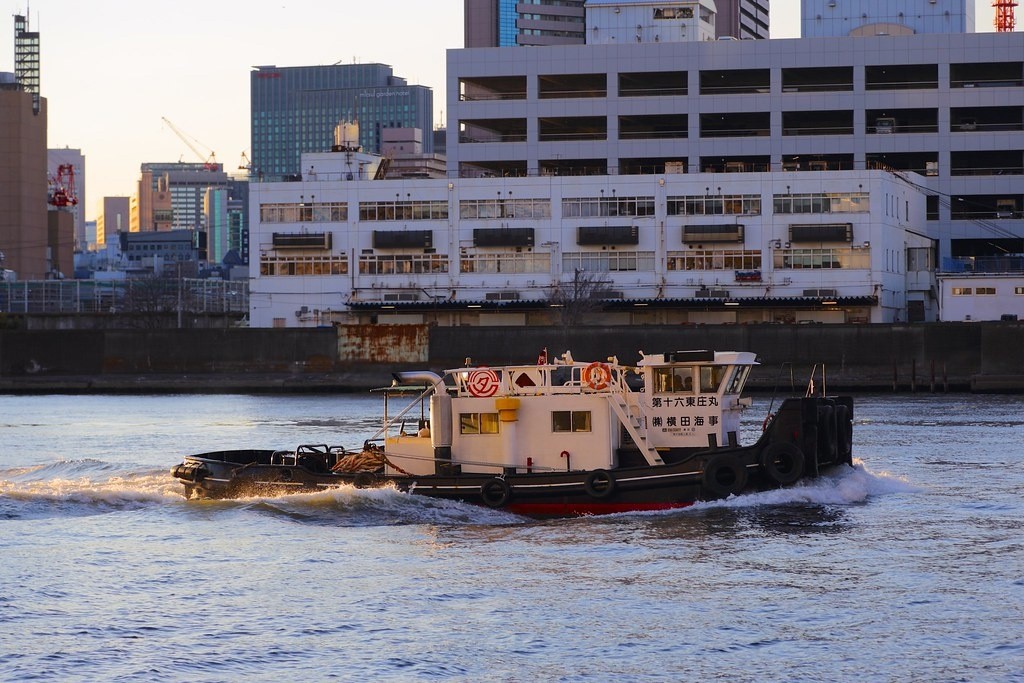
left=176, top=352, right=857, bottom=522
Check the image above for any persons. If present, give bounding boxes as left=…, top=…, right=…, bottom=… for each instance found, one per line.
left=667, top=374, right=692, bottom=391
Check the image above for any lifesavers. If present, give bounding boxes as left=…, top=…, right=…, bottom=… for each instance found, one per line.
left=760, top=439, right=807, bottom=486
left=821, top=404, right=837, bottom=456
left=836, top=405, right=851, bottom=455
left=353, top=471, right=379, bottom=490
left=584, top=467, right=616, bottom=499
left=702, top=453, right=750, bottom=497
left=584, top=362, right=612, bottom=390
left=481, top=477, right=511, bottom=509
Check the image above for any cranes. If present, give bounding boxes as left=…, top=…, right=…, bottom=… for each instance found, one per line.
left=162, top=116, right=219, bottom=170
